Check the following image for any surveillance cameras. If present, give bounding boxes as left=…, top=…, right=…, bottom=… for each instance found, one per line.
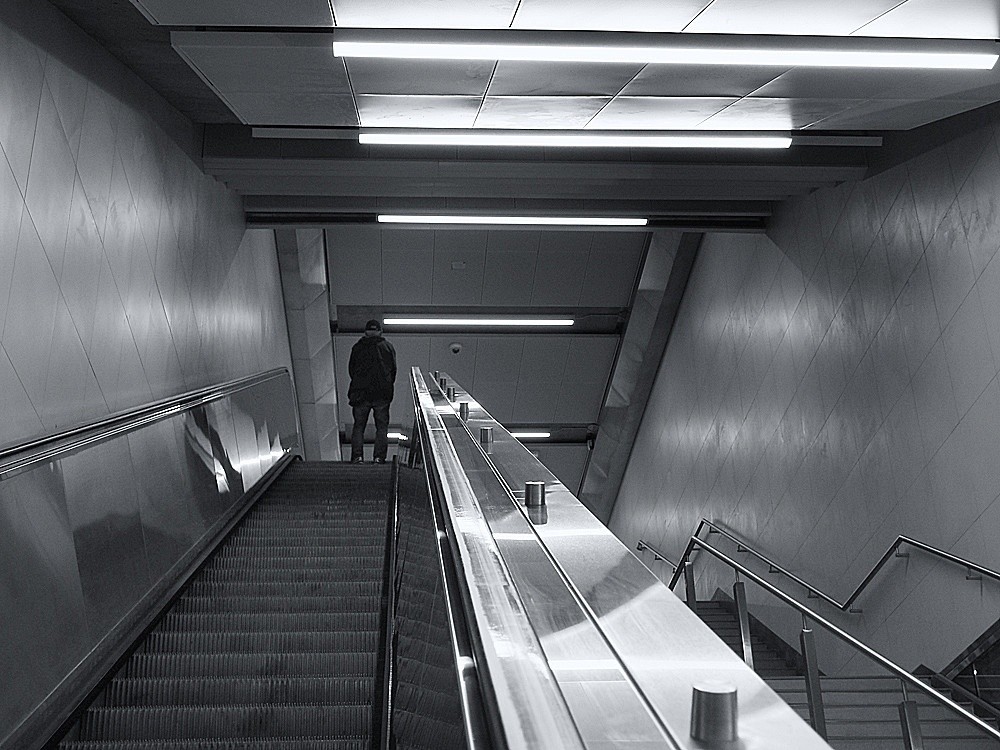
left=450, top=343, right=462, bottom=355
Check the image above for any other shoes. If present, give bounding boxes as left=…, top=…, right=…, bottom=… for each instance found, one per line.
left=374, top=457, right=387, bottom=465
left=353, top=457, right=363, bottom=465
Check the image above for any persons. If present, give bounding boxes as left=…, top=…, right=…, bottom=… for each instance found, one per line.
left=347, top=320, right=397, bottom=463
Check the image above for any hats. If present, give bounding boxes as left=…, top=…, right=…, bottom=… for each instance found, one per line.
left=365, top=319, right=381, bottom=331
